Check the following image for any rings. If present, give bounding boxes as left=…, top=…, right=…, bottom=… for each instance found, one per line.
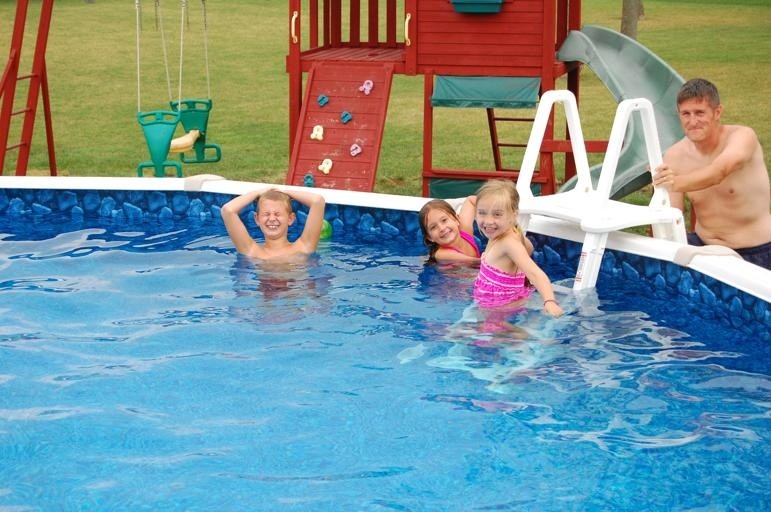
left=664, top=177, right=666, bottom=181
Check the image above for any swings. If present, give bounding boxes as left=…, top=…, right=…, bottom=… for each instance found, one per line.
left=132, top=1, right=221, bottom=178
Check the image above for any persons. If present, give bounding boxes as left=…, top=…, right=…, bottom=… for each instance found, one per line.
left=647, top=78, right=771, bottom=270
left=418, top=195, right=481, bottom=270
left=471, top=180, right=563, bottom=318
left=221, top=189, right=325, bottom=264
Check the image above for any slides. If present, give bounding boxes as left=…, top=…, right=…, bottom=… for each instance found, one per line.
left=552, top=21, right=686, bottom=200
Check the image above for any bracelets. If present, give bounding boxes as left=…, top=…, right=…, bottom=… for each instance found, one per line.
left=544, top=299, right=558, bottom=306
